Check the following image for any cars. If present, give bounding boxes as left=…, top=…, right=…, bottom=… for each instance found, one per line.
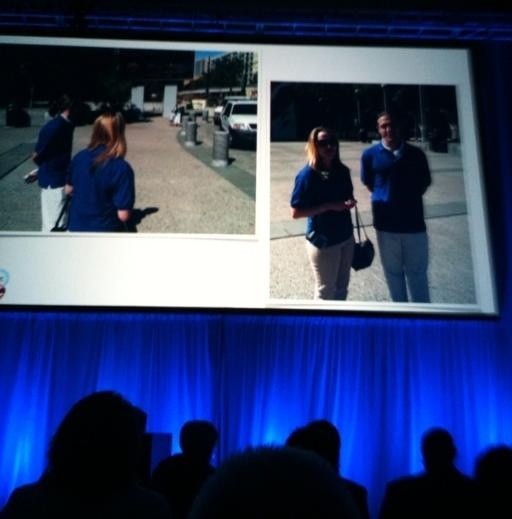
left=214, top=95, right=257, bottom=147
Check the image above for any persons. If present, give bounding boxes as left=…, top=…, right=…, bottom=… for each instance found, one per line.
left=361, top=109, right=433, bottom=303
left=30, top=91, right=76, bottom=233
left=0, top=385, right=510, bottom=518
left=290, top=125, right=358, bottom=301
left=63, top=110, right=146, bottom=230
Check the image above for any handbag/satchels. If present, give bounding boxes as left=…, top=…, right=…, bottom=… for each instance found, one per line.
left=352, top=238, right=375, bottom=272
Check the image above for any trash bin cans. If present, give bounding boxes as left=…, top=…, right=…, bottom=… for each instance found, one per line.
left=182, top=111, right=197, bottom=146
left=212, top=124, right=229, bottom=167
